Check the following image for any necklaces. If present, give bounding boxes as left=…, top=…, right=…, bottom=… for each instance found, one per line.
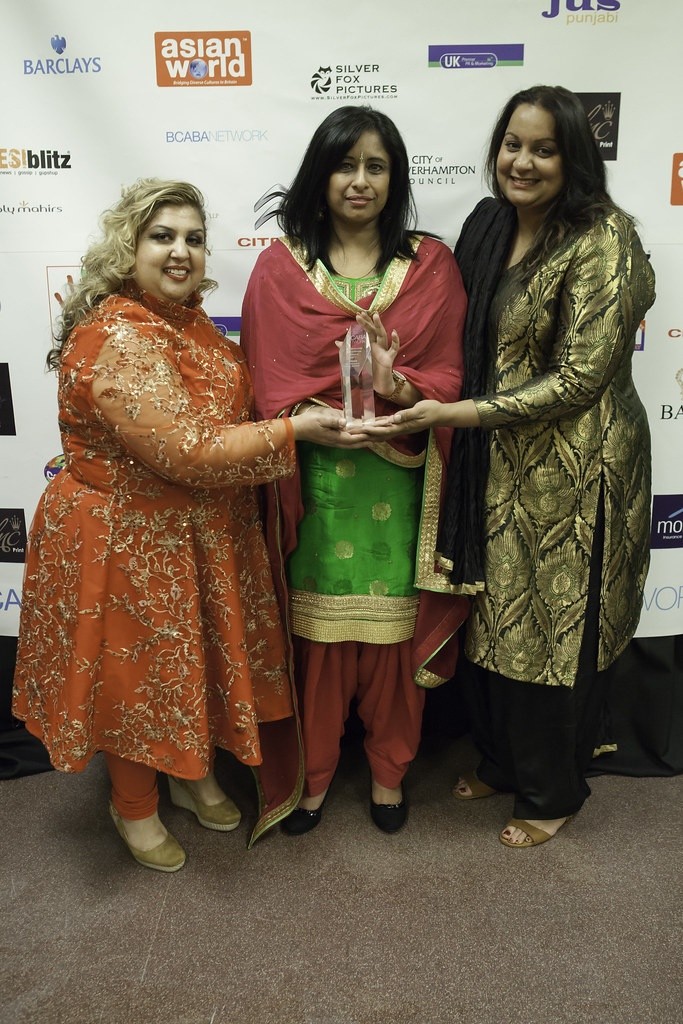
left=333, top=261, right=378, bottom=280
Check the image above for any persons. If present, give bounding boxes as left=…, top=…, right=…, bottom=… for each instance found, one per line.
left=242, top=107, right=468, bottom=836
left=11, top=175, right=372, bottom=872
left=347, top=85, right=657, bottom=849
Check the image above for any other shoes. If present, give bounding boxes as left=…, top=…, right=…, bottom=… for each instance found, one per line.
left=369, top=768, right=407, bottom=833
left=280, top=790, right=328, bottom=834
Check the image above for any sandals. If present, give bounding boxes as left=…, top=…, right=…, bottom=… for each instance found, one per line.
left=499, top=819, right=551, bottom=846
left=452, top=773, right=495, bottom=800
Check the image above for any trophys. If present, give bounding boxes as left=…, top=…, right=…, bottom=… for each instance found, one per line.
left=339, top=321, right=375, bottom=427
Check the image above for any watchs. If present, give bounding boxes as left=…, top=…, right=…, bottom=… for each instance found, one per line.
left=377, top=369, right=407, bottom=402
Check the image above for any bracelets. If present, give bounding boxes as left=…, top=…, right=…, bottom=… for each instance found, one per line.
left=291, top=401, right=303, bottom=417
left=305, top=404, right=316, bottom=412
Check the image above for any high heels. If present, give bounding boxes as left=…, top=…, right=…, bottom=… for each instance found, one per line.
left=168, top=767, right=240, bottom=831
left=108, top=796, right=186, bottom=871
